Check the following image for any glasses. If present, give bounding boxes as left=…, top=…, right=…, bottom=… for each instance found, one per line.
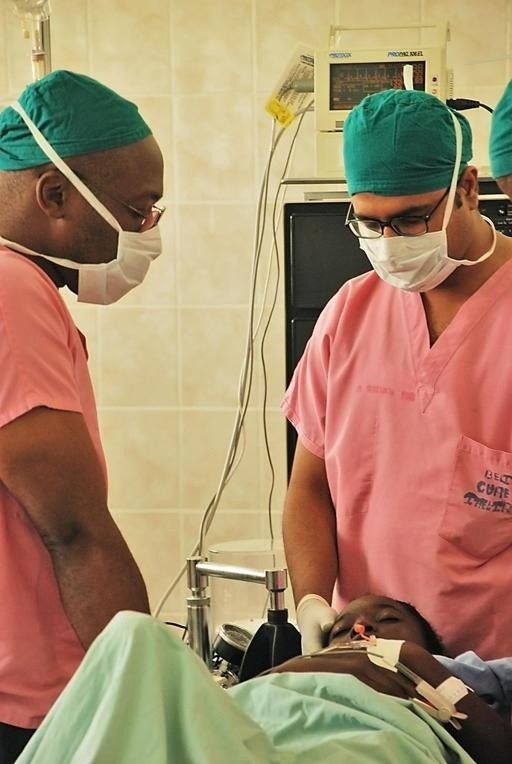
left=39, top=167, right=166, bottom=228
left=344, top=186, right=450, bottom=239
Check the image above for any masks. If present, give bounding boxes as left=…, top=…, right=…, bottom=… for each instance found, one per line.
left=358, top=183, right=461, bottom=293
left=64, top=170, right=162, bottom=305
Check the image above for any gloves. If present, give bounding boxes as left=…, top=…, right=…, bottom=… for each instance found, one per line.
left=296, top=594, right=338, bottom=656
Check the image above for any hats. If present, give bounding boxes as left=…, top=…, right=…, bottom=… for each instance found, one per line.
left=343, top=89, right=472, bottom=196
left=0, top=70, right=152, bottom=171
left=489, top=80, right=512, bottom=179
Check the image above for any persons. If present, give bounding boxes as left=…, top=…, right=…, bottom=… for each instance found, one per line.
left=278, top=87, right=510, bottom=716
left=488, top=81, right=512, bottom=198
left=0, top=70, right=166, bottom=763
left=279, top=596, right=512, bottom=763
